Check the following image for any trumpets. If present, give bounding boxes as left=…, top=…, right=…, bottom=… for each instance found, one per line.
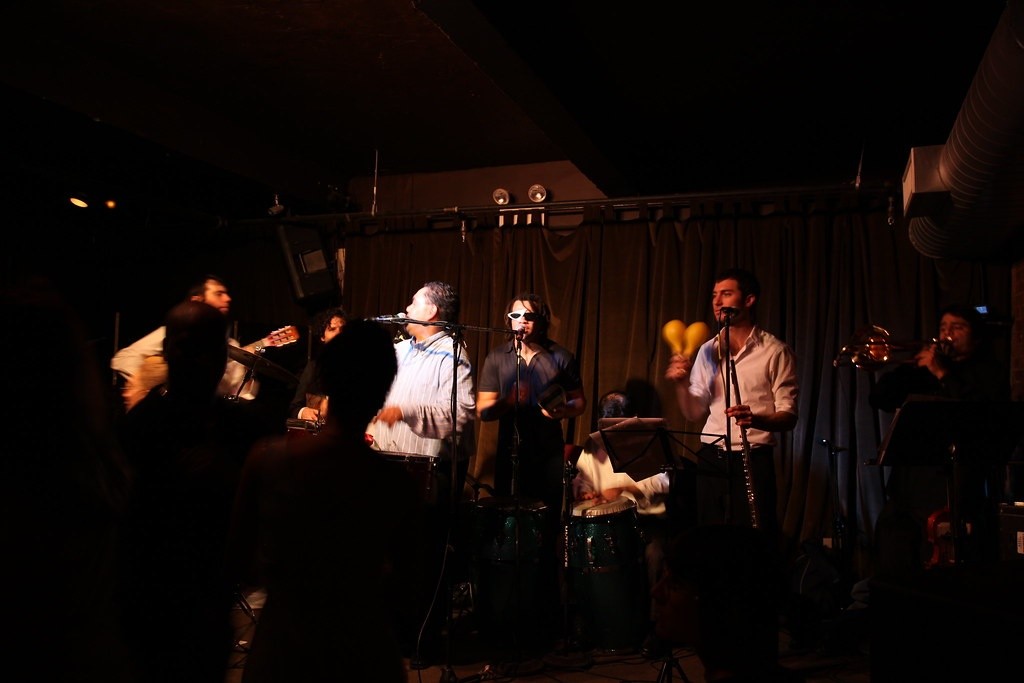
left=833, top=325, right=954, bottom=372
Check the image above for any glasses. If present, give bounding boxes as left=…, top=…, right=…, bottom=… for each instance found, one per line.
left=508, top=312, right=538, bottom=320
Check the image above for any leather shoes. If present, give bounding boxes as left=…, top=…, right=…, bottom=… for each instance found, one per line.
left=567, top=635, right=593, bottom=652
left=639, top=631, right=666, bottom=659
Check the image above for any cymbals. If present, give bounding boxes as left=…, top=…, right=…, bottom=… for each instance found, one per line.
left=226, top=340, right=298, bottom=388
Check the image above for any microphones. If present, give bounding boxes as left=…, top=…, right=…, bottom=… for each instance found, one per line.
left=515, top=325, right=524, bottom=340
left=365, top=312, right=406, bottom=324
left=720, top=307, right=740, bottom=318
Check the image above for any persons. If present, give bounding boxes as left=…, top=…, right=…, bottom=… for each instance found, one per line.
left=0, top=266, right=477, bottom=683
left=477, top=294, right=587, bottom=498
left=666, top=269, right=802, bottom=683
left=568, top=390, right=677, bottom=659
left=880, top=306, right=1010, bottom=496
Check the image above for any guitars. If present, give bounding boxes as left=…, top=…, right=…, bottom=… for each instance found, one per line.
left=123, top=324, right=301, bottom=410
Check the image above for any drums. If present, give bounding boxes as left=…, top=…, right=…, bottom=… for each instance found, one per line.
left=569, top=496, right=646, bottom=573
left=475, top=495, right=548, bottom=571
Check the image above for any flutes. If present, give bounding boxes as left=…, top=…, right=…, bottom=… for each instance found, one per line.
left=725, top=350, right=761, bottom=532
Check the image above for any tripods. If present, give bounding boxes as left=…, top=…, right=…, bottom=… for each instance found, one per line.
left=601, top=429, right=734, bottom=683
